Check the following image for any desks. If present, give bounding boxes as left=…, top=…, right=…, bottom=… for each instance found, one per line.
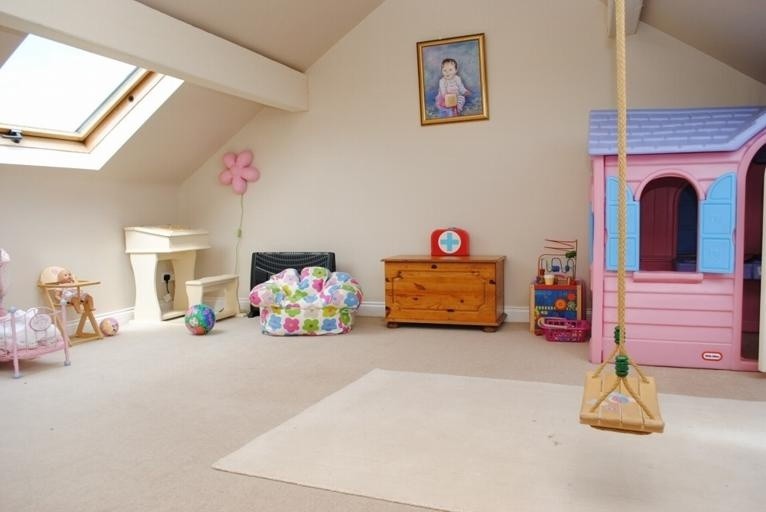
left=124, top=226, right=210, bottom=322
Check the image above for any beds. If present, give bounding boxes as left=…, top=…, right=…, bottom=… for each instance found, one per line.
left=0, top=296, right=72, bottom=379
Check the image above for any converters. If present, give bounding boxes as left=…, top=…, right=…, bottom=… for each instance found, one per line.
left=164, top=274, right=169, bottom=279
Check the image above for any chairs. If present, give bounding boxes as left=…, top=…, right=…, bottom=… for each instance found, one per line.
left=247, top=265, right=364, bottom=337
left=36, top=266, right=103, bottom=346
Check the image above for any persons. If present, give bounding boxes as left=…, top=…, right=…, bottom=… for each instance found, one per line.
left=56, top=269, right=95, bottom=313
left=433, top=58, right=471, bottom=116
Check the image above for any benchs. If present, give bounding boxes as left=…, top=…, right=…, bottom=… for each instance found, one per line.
left=184, top=272, right=242, bottom=322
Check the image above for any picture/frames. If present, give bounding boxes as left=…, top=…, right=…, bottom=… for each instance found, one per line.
left=416, top=33, right=489, bottom=127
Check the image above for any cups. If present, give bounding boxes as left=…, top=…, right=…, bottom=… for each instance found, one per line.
left=544, top=275, right=554, bottom=286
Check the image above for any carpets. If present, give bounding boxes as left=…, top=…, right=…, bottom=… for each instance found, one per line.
left=211, top=366, right=765, bottom=511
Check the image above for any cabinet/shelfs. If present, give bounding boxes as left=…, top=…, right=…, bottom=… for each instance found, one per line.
left=380, top=255, right=508, bottom=333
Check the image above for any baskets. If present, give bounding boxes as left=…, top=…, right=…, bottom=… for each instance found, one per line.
left=538, top=317, right=590, bottom=343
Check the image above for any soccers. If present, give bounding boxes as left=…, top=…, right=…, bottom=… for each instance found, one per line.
left=100, top=318, right=118, bottom=335
left=185, top=305, right=216, bottom=335
